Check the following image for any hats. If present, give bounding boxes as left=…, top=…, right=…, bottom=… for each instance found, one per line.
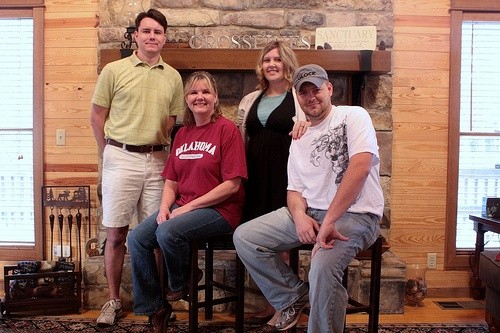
left=292, top=64, right=329, bottom=93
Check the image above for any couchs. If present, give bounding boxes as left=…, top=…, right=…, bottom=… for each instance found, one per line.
left=480, top=252, right=500, bottom=333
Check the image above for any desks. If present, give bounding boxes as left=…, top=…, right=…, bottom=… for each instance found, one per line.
left=469, top=215, right=500, bottom=299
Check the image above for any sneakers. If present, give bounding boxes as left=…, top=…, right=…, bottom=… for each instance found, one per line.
left=273, top=291, right=310, bottom=331
left=146, top=311, right=177, bottom=323
left=95, top=298, right=124, bottom=327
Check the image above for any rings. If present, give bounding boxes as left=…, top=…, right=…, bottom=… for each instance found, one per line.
left=301, top=125, right=304, bottom=128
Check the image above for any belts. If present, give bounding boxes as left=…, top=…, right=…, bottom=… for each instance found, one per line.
left=108, top=139, right=165, bottom=153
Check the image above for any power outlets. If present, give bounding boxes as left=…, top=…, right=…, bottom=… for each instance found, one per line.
left=427, top=253, right=437, bottom=269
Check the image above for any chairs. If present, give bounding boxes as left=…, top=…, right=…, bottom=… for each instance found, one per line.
left=3, top=265, right=83, bottom=317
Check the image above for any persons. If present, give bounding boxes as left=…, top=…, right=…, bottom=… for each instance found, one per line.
left=231, top=41, right=384, bottom=333
left=91, top=9, right=184, bottom=326
left=127, top=72, right=248, bottom=333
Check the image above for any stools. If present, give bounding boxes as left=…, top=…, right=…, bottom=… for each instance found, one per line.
left=148, top=232, right=383, bottom=333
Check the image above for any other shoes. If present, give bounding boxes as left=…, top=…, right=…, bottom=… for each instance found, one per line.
left=150, top=301, right=173, bottom=333
left=166, top=268, right=203, bottom=302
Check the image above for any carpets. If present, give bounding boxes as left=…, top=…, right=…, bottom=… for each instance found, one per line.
left=0, top=318, right=491, bottom=333
